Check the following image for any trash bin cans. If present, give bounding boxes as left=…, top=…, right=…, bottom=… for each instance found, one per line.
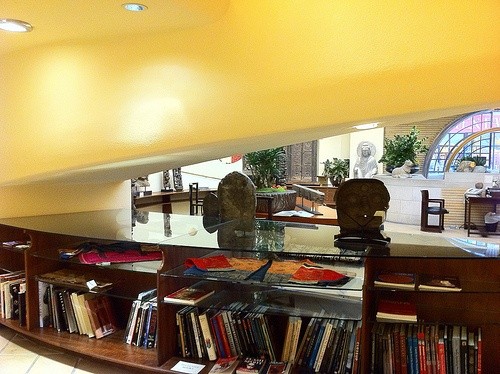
left=485, top=213, right=500, bottom=232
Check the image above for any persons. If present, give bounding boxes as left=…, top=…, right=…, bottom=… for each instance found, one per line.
left=353, top=143, right=378, bottom=179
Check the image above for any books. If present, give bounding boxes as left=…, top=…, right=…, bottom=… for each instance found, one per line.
left=0, top=268, right=483, bottom=374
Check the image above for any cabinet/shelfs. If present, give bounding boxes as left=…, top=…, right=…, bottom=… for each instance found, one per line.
left=0, top=209, right=500, bottom=374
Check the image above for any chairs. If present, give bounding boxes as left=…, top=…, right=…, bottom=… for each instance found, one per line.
left=189, top=183, right=205, bottom=216
left=419, top=189, right=449, bottom=233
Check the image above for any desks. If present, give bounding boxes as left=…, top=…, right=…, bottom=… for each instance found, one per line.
left=464, top=193, right=500, bottom=238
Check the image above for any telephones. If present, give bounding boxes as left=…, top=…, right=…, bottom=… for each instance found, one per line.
left=465, top=188, right=484, bottom=195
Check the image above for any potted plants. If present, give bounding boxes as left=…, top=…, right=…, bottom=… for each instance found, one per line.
left=243, top=145, right=297, bottom=211
left=323, top=157, right=349, bottom=186
left=376, top=125, right=430, bottom=174
left=473, top=155, right=486, bottom=172
left=460, top=156, right=474, bottom=169
